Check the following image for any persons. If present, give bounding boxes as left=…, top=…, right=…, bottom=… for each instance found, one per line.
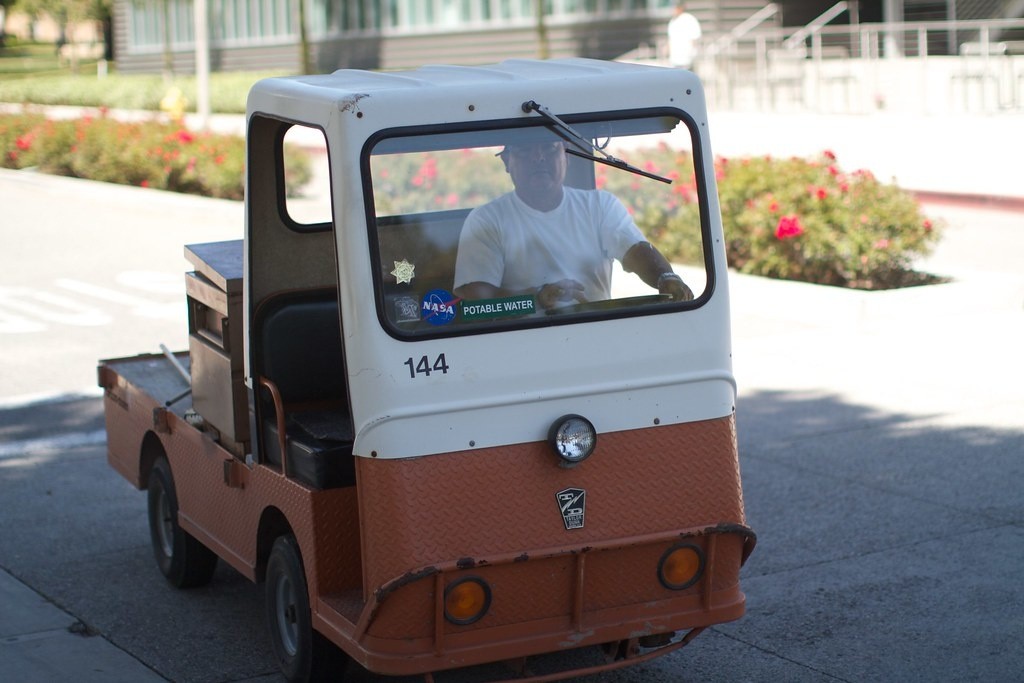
left=667, top=3, right=702, bottom=70
left=451, top=141, right=694, bottom=323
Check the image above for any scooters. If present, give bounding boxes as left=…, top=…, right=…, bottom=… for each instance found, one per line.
left=97, top=57, right=757, bottom=683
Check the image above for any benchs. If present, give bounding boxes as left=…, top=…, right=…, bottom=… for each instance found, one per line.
left=253, top=278, right=454, bottom=491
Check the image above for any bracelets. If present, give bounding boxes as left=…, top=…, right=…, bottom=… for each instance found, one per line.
left=536, top=283, right=548, bottom=295
left=657, top=272, right=682, bottom=290
